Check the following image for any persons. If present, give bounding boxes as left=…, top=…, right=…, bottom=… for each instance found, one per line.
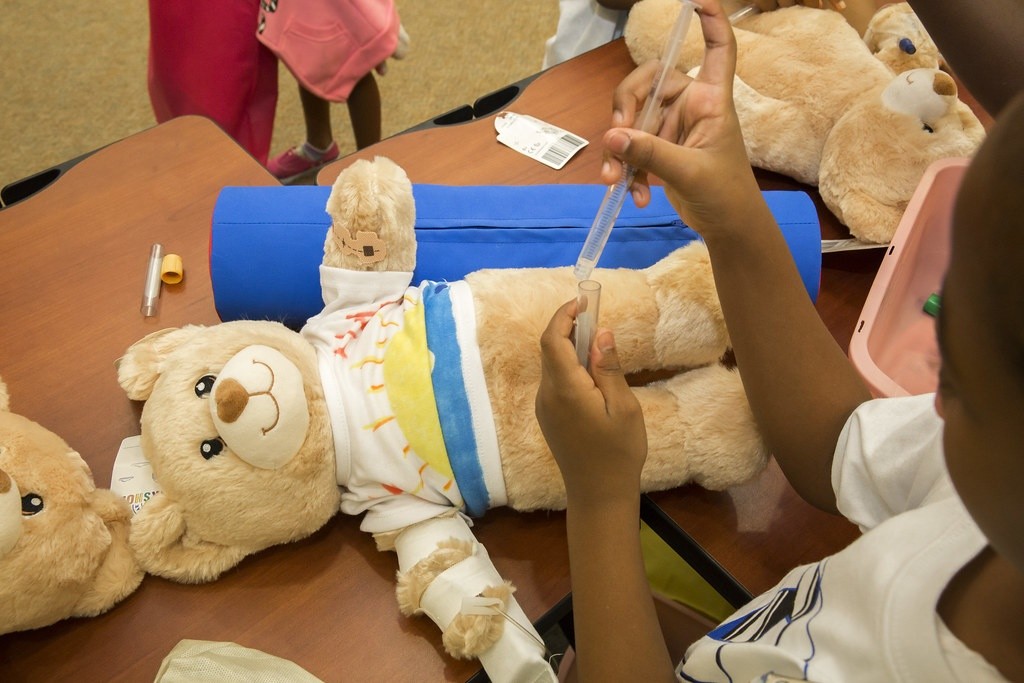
left=266, top=71, right=382, bottom=183
left=535, top=92, right=1024, bottom=683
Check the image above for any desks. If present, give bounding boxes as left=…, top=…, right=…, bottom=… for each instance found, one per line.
left=312, top=0, right=995, bottom=602
left=0, top=114, right=739, bottom=683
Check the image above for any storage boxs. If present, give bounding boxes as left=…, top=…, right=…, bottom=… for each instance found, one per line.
left=847, top=157, right=971, bottom=398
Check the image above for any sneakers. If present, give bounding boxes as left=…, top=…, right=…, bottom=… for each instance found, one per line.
left=266, top=137, right=340, bottom=187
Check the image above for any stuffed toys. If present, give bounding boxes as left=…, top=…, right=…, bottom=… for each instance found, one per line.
left=119, top=154, right=767, bottom=683
left=0, top=379, right=146, bottom=636
left=624, top=0, right=986, bottom=242
